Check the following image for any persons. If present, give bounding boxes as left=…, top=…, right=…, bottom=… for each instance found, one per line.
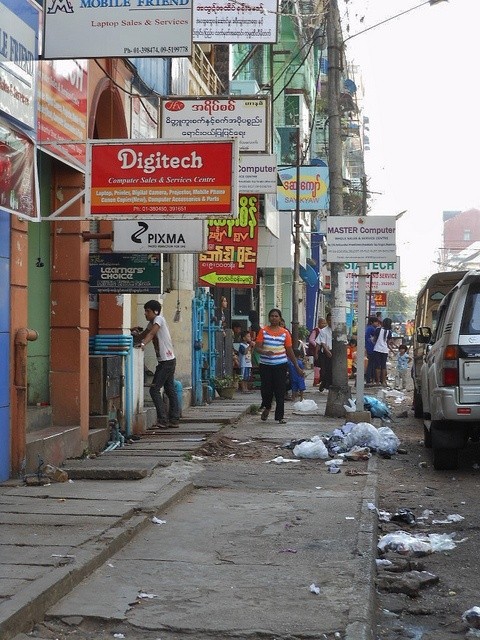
left=287, top=349, right=306, bottom=402
left=365, top=317, right=379, bottom=387
left=239, top=331, right=255, bottom=393
left=345, top=339, right=357, bottom=361
left=256, top=309, right=304, bottom=424
left=133, top=299, right=179, bottom=429
left=316, top=313, right=332, bottom=392
left=309, top=318, right=327, bottom=387
left=231, top=322, right=241, bottom=368
left=216, top=296, right=228, bottom=338
left=373, top=318, right=396, bottom=387
left=396, top=345, right=412, bottom=389
left=280, top=319, right=285, bottom=327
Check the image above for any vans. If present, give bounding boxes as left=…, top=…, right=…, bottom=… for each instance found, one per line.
left=409, top=271, right=470, bottom=420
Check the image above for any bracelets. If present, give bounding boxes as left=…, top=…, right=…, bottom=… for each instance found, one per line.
left=139, top=340, right=144, bottom=347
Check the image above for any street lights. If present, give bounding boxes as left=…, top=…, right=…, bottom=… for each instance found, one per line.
left=320, top=0, right=447, bottom=418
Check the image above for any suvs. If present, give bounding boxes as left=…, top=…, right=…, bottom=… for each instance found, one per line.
left=416, top=268, right=480, bottom=472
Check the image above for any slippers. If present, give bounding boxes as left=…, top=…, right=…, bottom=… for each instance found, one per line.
left=169, top=423, right=179, bottom=427
left=148, top=423, right=167, bottom=431
left=261, top=409, right=270, bottom=420
left=275, top=417, right=287, bottom=423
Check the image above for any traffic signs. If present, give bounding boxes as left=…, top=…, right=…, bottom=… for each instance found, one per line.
left=349, top=207, right=408, bottom=337
